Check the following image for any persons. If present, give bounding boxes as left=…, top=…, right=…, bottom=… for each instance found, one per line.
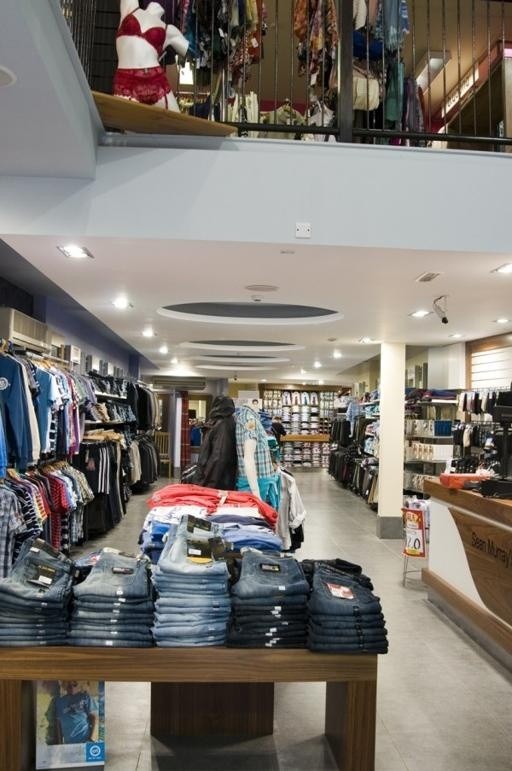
left=112, top=0, right=197, bottom=113
left=190, top=395, right=237, bottom=490
left=232, top=403, right=274, bottom=503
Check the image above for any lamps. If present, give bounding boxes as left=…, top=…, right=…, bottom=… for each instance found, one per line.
left=432, top=296, right=449, bottom=324
left=408, top=50, right=452, bottom=95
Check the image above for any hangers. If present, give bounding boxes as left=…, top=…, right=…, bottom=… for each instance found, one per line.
left=457, top=386, right=512, bottom=393
left=81, top=429, right=124, bottom=443
left=456, top=420, right=512, bottom=435
left=1, top=338, right=78, bottom=374
left=1, top=459, right=71, bottom=486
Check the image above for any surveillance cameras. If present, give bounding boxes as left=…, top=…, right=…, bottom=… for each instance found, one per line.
left=433, top=303, right=449, bottom=324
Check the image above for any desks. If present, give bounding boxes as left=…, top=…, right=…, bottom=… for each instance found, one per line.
left=150, top=682, right=274, bottom=749
left=0, top=644, right=376, bottom=770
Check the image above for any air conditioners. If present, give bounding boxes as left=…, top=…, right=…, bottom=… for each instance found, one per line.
left=0, top=307, right=52, bottom=355
left=151, top=377, right=206, bottom=390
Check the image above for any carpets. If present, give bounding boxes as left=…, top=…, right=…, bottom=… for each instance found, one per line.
left=133, top=433, right=150, bottom=442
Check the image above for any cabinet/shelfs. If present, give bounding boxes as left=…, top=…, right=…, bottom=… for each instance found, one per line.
left=259, top=383, right=353, bottom=472
left=80, top=391, right=160, bottom=539
left=355, top=398, right=461, bottom=530
